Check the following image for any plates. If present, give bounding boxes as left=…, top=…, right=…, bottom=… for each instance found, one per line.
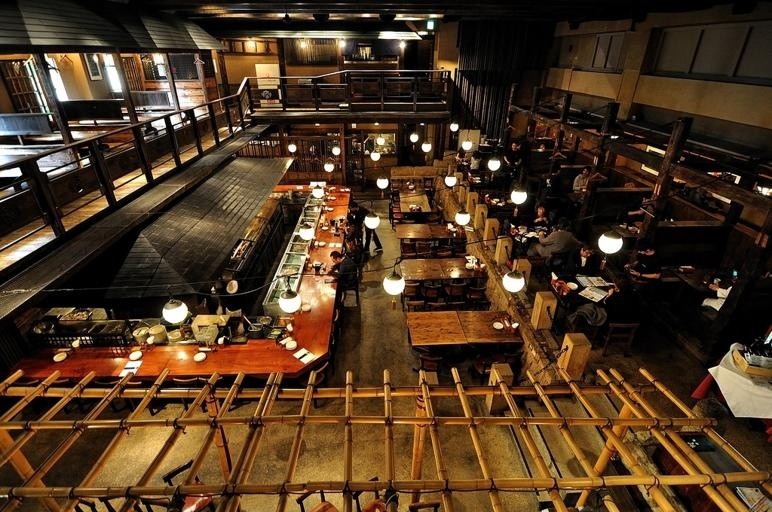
left=128, top=350, right=143, bottom=361
left=53, top=352, right=67, bottom=362
left=450, top=228, right=457, bottom=232
left=302, top=304, right=311, bottom=312
left=318, top=242, right=326, bottom=247
left=192, top=351, right=207, bottom=362
left=132, top=325, right=183, bottom=345
left=286, top=341, right=298, bottom=350
left=330, top=197, right=337, bottom=200
left=493, top=322, right=504, bottom=330
left=567, top=282, right=578, bottom=290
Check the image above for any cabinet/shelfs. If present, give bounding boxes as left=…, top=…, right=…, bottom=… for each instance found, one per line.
left=339, top=55, right=400, bottom=83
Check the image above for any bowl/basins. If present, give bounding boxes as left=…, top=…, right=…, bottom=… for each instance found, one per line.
left=465, top=263, right=473, bottom=269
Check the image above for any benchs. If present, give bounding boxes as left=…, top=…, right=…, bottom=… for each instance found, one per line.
left=0, top=91, right=176, bottom=184
left=596, top=187, right=772, bottom=359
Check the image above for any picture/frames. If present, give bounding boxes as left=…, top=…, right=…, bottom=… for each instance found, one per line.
left=84, top=53, right=104, bottom=81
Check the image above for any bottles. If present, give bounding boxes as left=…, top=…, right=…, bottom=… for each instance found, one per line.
left=351, top=48, right=367, bottom=60
left=325, top=218, right=329, bottom=227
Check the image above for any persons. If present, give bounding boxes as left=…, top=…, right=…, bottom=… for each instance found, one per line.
left=343, top=215, right=356, bottom=249
left=328, top=251, right=356, bottom=302
left=349, top=202, right=382, bottom=250
left=456, top=142, right=738, bottom=323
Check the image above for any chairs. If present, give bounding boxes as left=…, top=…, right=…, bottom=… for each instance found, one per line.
left=475, top=355, right=507, bottom=386
left=73, top=460, right=445, bottom=512
left=12, top=330, right=337, bottom=416
left=413, top=354, right=442, bottom=375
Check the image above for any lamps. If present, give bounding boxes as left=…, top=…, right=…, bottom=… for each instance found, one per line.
left=364, top=211, right=380, bottom=229
left=510, top=186, right=527, bottom=205
left=487, top=155, right=501, bottom=172
left=278, top=275, right=302, bottom=314
left=461, top=138, right=473, bottom=151
left=312, top=185, right=324, bottom=199
left=376, top=136, right=385, bottom=146
left=323, top=161, right=335, bottom=173
left=370, top=149, right=381, bottom=162
left=409, top=133, right=419, bottom=143
left=162, top=298, right=189, bottom=323
left=597, top=230, right=624, bottom=255
left=502, top=268, right=526, bottom=293
left=376, top=175, right=389, bottom=190
left=449, top=124, right=459, bottom=132
left=454, top=206, right=470, bottom=226
left=444, top=174, right=457, bottom=188
left=332, top=146, right=341, bottom=156
left=421, top=141, right=432, bottom=153
left=382, top=264, right=406, bottom=296
left=299, top=223, right=315, bottom=241
left=287, top=143, right=297, bottom=153
